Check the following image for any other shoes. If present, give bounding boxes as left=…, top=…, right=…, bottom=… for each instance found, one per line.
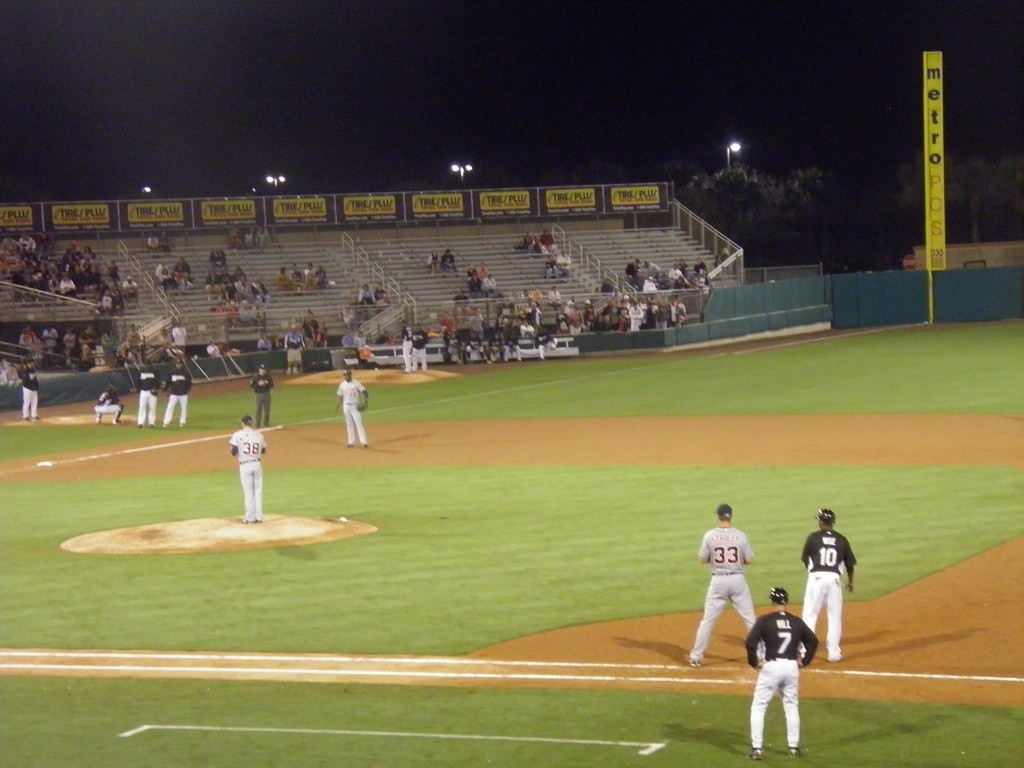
left=179, top=423, right=185, bottom=428
left=162, top=424, right=168, bottom=428
left=689, top=659, right=701, bottom=667
left=138, top=424, right=143, bottom=428
left=747, top=748, right=763, bottom=760
left=827, top=656, right=845, bottom=662
left=150, top=424, right=154, bottom=428
left=362, top=444, right=367, bottom=448
left=113, top=419, right=123, bottom=425
left=96, top=417, right=103, bottom=425
left=348, top=444, right=354, bottom=448
left=23, top=416, right=42, bottom=423
left=242, top=519, right=263, bottom=524
left=789, top=746, right=800, bottom=758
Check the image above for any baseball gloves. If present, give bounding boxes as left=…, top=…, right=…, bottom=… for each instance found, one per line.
left=151, top=388, right=158, bottom=395
left=357, top=404, right=366, bottom=412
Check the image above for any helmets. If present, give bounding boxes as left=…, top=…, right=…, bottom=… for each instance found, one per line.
left=815, top=508, right=836, bottom=525
left=770, top=586, right=788, bottom=605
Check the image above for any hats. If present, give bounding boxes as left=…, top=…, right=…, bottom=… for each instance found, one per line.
left=342, top=368, right=352, bottom=375
left=258, top=363, right=266, bottom=369
left=238, top=415, right=252, bottom=425
left=567, top=300, right=574, bottom=306
left=127, top=275, right=132, bottom=280
left=175, top=359, right=182, bottom=364
left=400, top=320, right=406, bottom=324
left=715, top=503, right=732, bottom=519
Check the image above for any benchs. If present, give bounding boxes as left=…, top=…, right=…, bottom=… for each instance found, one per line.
left=0, top=225, right=718, bottom=366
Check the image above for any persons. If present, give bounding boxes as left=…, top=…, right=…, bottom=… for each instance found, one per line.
left=249, top=363, right=275, bottom=429
left=0, top=223, right=715, bottom=375
left=133, top=357, right=161, bottom=429
left=334, top=369, right=369, bottom=449
left=161, top=357, right=194, bottom=428
left=743, top=587, right=820, bottom=761
left=93, top=383, right=126, bottom=426
left=16, top=357, right=41, bottom=423
left=797, top=507, right=857, bottom=663
left=688, top=503, right=766, bottom=669
left=228, top=415, right=267, bottom=524
